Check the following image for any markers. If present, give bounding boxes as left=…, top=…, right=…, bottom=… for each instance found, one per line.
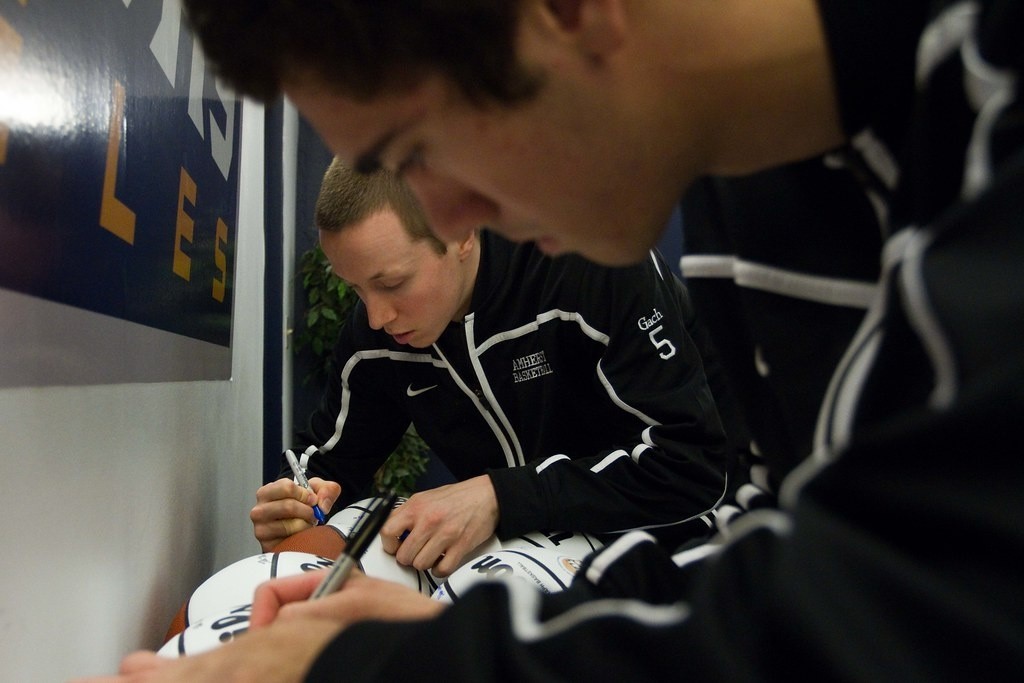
left=309, top=487, right=396, bottom=602
left=286, top=449, right=325, bottom=522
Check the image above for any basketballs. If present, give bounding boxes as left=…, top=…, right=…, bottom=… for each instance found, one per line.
left=153, top=496, right=606, bottom=659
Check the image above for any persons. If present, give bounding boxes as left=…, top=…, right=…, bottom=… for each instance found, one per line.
left=71, top=0, right=1020, bottom=683
